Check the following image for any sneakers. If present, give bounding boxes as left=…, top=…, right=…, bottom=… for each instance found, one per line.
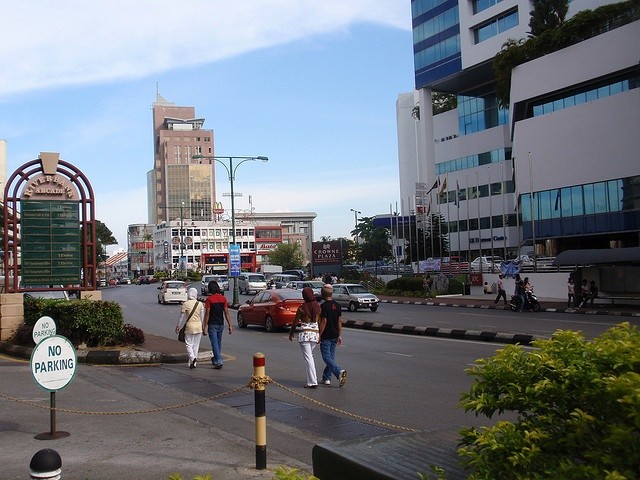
left=338, top=369, right=347, bottom=387
left=319, top=378, right=331, bottom=385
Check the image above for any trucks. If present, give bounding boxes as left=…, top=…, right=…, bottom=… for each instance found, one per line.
left=255, top=265, right=282, bottom=273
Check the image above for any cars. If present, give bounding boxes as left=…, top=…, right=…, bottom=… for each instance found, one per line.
left=282, top=281, right=322, bottom=297
left=237, top=289, right=306, bottom=332
left=136, top=276, right=150, bottom=285
left=471, top=256, right=505, bottom=273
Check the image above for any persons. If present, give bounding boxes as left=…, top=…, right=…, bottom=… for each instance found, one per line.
left=567, top=278, right=579, bottom=310
left=318, top=283, right=347, bottom=388
left=296, top=272, right=345, bottom=283
left=289, top=287, right=322, bottom=388
left=484, top=282, right=492, bottom=294
left=175, top=288, right=206, bottom=369
left=202, top=280, right=233, bottom=369
left=579, top=279, right=598, bottom=310
left=511, top=273, right=534, bottom=313
left=494, top=274, right=510, bottom=304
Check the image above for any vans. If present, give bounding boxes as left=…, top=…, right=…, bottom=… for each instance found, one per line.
left=269, top=274, right=299, bottom=287
left=201, top=275, right=225, bottom=296
left=220, top=275, right=229, bottom=290
left=0, top=276, right=69, bottom=300
left=239, top=272, right=267, bottom=295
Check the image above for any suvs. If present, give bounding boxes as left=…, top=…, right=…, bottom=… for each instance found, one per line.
left=157, top=281, right=187, bottom=305
left=331, top=284, right=379, bottom=312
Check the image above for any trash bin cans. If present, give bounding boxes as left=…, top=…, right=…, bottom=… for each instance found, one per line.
left=462, top=281, right=471, bottom=295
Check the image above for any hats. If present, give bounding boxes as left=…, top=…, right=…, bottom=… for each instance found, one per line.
left=321, top=283, right=334, bottom=296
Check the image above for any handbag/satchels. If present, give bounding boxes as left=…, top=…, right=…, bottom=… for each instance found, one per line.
left=178, top=324, right=186, bottom=343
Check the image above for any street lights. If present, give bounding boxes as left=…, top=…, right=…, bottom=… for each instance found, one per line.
left=159, top=207, right=204, bottom=257
left=192, top=154, right=269, bottom=309
left=350, top=209, right=361, bottom=243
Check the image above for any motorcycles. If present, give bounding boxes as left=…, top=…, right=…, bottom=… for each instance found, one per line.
left=510, top=285, right=540, bottom=312
